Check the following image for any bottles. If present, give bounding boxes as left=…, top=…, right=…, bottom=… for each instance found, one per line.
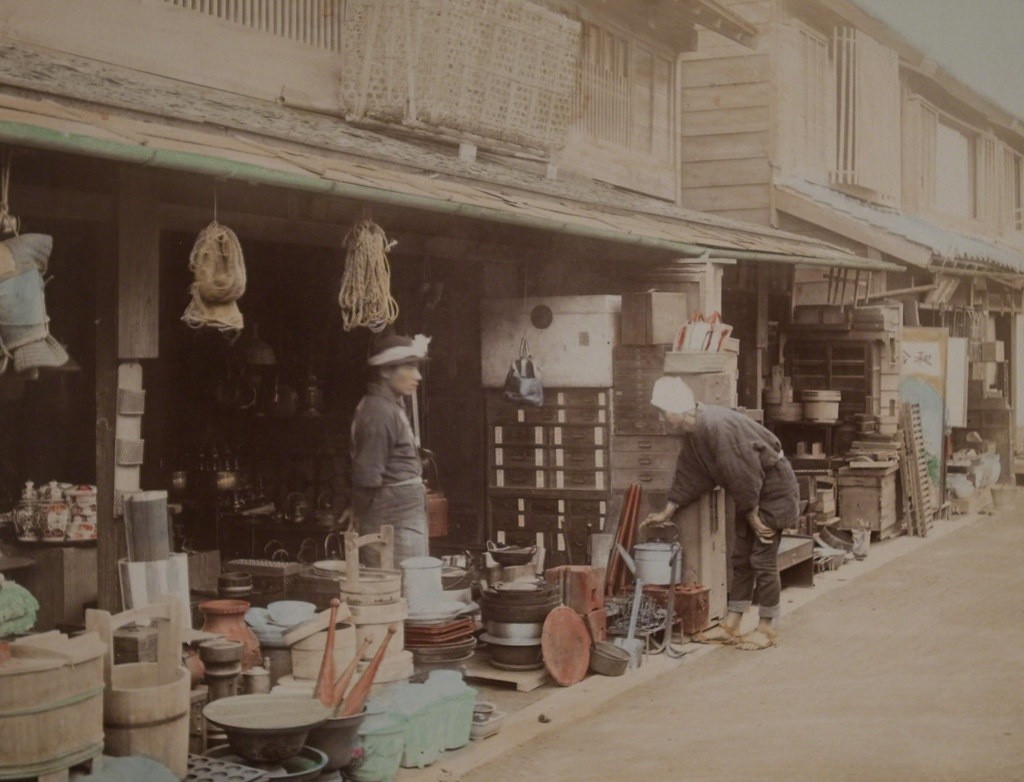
left=14, top=478, right=71, bottom=541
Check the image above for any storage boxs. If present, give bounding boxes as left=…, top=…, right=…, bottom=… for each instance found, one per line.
left=621, top=291, right=688, bottom=348
left=478, top=293, right=621, bottom=388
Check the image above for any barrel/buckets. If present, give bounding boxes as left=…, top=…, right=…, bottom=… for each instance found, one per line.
left=83, top=595, right=190, bottom=780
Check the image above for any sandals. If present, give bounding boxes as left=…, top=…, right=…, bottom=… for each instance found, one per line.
left=736, top=627, right=777, bottom=651
left=690, top=623, right=741, bottom=645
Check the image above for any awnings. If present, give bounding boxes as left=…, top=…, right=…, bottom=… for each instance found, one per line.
left=773, top=178, right=1024, bottom=278
left=0, top=84, right=906, bottom=272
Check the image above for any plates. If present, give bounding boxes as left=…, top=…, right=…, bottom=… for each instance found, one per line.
left=241, top=601, right=315, bottom=644
left=309, top=560, right=363, bottom=578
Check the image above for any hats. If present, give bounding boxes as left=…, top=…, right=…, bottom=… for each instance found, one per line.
left=650, top=377, right=695, bottom=413
left=367, top=334, right=433, bottom=365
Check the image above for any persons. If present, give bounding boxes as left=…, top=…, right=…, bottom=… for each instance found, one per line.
left=637, top=376, right=799, bottom=650
left=347, top=334, right=430, bottom=568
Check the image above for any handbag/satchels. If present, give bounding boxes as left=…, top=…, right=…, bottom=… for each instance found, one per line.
left=503, top=338, right=544, bottom=407
left=673, top=312, right=733, bottom=352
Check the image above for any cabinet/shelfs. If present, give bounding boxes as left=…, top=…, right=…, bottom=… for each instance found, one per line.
left=784, top=331, right=886, bottom=455
left=482, top=348, right=739, bottom=631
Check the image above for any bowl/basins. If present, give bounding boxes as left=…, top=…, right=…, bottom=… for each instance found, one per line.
left=204, top=695, right=328, bottom=761
left=589, top=641, right=630, bottom=676
left=407, top=638, right=474, bottom=685
left=267, top=602, right=316, bottom=624
left=318, top=693, right=370, bottom=771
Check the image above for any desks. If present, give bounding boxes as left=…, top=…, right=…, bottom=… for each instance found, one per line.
left=15, top=539, right=101, bottom=629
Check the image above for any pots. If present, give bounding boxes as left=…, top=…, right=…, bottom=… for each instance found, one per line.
left=487, top=540, right=538, bottom=565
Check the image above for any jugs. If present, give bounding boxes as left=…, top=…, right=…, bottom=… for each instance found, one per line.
left=615, top=519, right=683, bottom=586
